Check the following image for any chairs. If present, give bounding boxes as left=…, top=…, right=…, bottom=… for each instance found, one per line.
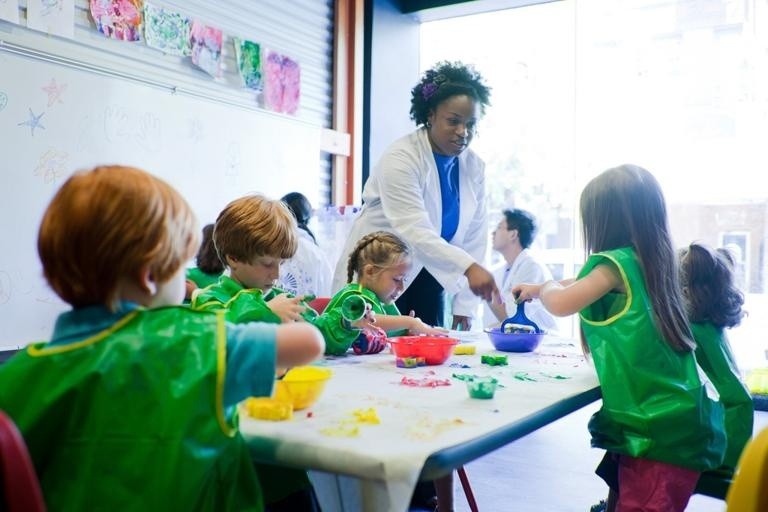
left=693, top=424, right=768, bottom=512
left=0, top=411, right=46, bottom=512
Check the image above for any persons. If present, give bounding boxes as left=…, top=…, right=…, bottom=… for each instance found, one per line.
left=192, top=196, right=375, bottom=358
left=483, top=207, right=557, bottom=330
left=676, top=240, right=753, bottom=483
left=510, top=166, right=726, bottom=512
left=185, top=225, right=228, bottom=289
left=331, top=60, right=503, bottom=331
left=0, top=165, right=325, bottom=512
left=182, top=193, right=334, bottom=301
left=321, top=231, right=449, bottom=339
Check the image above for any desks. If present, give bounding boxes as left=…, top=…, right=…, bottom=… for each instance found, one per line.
left=232, top=331, right=602, bottom=512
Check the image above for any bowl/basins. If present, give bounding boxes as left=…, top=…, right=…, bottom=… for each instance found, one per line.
left=483, top=328, right=546, bottom=353
left=386, top=336, right=459, bottom=366
left=273, top=366, right=334, bottom=410
